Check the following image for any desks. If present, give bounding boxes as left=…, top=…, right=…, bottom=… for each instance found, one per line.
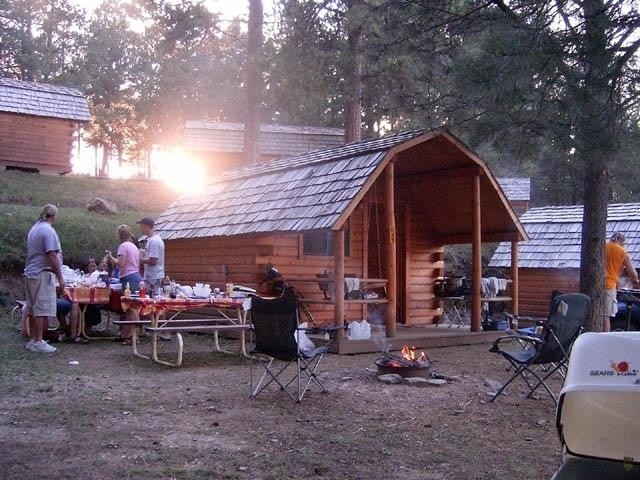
left=274, top=277, right=388, bottom=284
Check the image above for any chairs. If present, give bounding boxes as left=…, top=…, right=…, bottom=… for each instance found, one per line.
left=433, top=277, right=472, bottom=328
left=248, top=284, right=330, bottom=405
left=489, top=292, right=593, bottom=405
left=546, top=331, right=640, bottom=480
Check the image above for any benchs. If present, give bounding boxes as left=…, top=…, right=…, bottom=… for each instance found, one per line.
left=10, top=299, right=31, bottom=338
left=145, top=324, right=257, bottom=368
left=299, top=297, right=387, bottom=321
left=113, top=318, right=237, bottom=361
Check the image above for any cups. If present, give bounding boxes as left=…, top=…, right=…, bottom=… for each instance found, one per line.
left=158, top=288, right=163, bottom=298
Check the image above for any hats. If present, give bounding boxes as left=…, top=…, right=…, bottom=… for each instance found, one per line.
left=135, top=217, right=154, bottom=225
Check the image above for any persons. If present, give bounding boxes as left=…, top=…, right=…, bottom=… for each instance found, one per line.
left=602, top=233, right=640, bottom=333
left=24, top=204, right=171, bottom=353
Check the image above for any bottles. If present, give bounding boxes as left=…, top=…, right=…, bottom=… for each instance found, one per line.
left=170, top=279, right=176, bottom=298
left=139, top=279, right=146, bottom=299
left=225, top=274, right=234, bottom=297
left=124, top=282, right=131, bottom=297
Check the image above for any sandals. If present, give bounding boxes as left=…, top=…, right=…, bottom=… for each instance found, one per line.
left=121, top=335, right=141, bottom=345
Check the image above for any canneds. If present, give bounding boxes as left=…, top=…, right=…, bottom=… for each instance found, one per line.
left=214, top=288, right=220, bottom=299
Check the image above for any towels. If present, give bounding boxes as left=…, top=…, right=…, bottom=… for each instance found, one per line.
left=345, top=278, right=360, bottom=293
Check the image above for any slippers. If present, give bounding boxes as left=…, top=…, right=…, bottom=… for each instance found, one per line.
left=158, top=336, right=171, bottom=341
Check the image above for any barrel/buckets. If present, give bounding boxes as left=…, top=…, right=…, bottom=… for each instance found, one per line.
left=447, top=276, right=463, bottom=297
left=347, top=320, right=362, bottom=340
left=359, top=320, right=371, bottom=340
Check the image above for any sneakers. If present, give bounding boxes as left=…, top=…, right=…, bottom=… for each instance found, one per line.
left=25, top=339, right=56, bottom=353
